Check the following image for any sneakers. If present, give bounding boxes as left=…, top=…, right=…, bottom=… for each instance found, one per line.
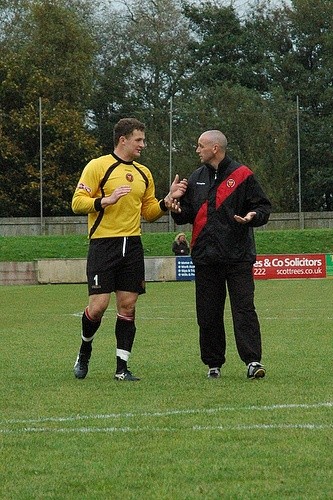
left=207, top=367, right=221, bottom=378
left=246, top=361, right=266, bottom=380
left=74, top=347, right=92, bottom=379
left=113, top=367, right=140, bottom=382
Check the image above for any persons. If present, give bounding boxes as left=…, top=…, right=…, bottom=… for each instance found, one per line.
left=172, top=232, right=190, bottom=256
left=72, top=118, right=188, bottom=381
left=164, top=130, right=273, bottom=379
